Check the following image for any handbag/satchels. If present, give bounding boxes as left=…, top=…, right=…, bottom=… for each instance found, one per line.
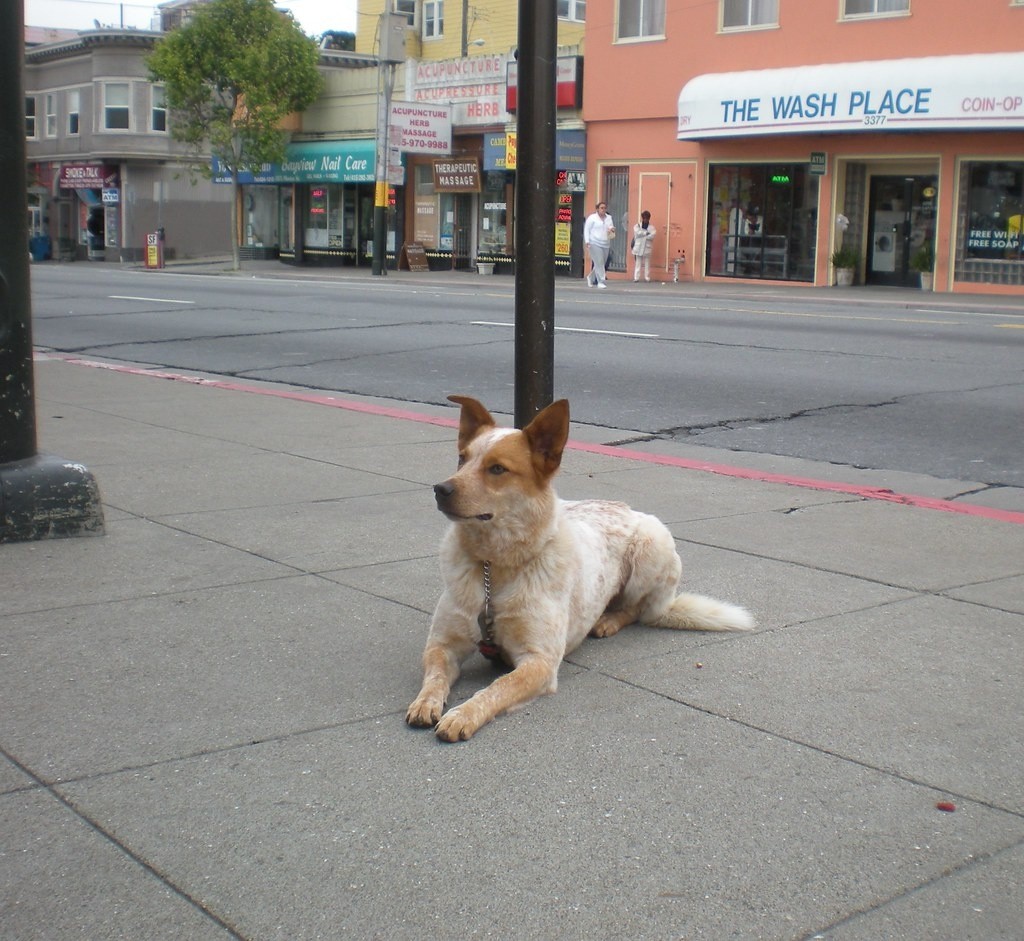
left=630, top=239, right=635, bottom=248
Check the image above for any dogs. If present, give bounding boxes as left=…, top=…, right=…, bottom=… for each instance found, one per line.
left=403, top=394, right=758, bottom=743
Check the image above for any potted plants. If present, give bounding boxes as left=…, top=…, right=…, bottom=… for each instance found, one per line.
left=919, top=242, right=935, bottom=292
left=476, top=253, right=496, bottom=276
left=830, top=247, right=859, bottom=287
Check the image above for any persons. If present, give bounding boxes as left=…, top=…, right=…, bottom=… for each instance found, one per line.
left=632, top=210, right=656, bottom=281
left=584, top=203, right=614, bottom=288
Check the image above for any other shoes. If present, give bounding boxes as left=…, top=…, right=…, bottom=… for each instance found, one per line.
left=587, top=276, right=593, bottom=288
left=597, top=283, right=606, bottom=289
left=634, top=277, right=651, bottom=282
